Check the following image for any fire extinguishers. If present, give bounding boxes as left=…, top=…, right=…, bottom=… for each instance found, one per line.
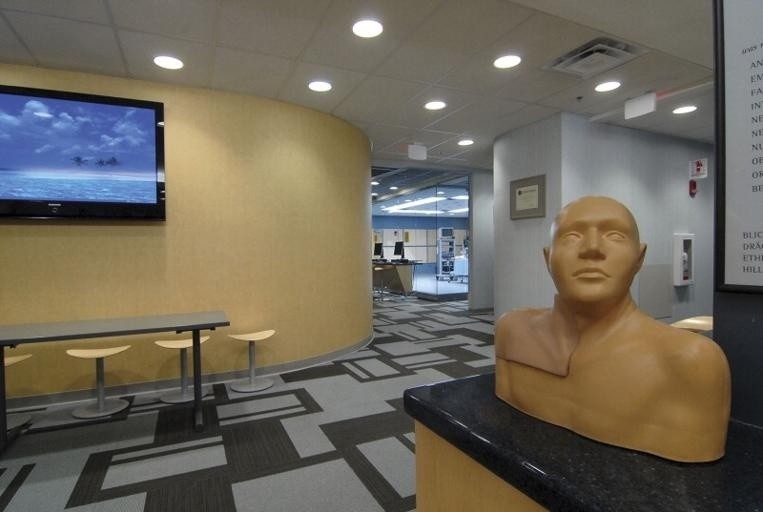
left=683, top=249, right=690, bottom=280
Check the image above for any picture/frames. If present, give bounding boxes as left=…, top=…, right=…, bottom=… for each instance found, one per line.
left=712, top=0, right=762, bottom=295
left=508, top=174, right=545, bottom=221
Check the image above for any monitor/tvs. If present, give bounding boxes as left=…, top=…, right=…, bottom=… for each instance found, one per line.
left=373, top=243, right=384, bottom=258
left=438, top=227, right=454, bottom=239
left=0, top=84, right=167, bottom=218
left=394, top=241, right=405, bottom=258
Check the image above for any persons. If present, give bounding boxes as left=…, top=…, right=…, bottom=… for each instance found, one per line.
left=493, top=194, right=731, bottom=463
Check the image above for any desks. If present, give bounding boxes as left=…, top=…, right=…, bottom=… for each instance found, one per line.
left=0, top=307, right=231, bottom=456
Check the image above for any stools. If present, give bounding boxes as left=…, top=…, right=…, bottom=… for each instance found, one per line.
left=3, top=353, right=33, bottom=433
left=153, top=336, right=210, bottom=405
left=65, top=344, right=132, bottom=420
left=228, top=328, right=276, bottom=393
